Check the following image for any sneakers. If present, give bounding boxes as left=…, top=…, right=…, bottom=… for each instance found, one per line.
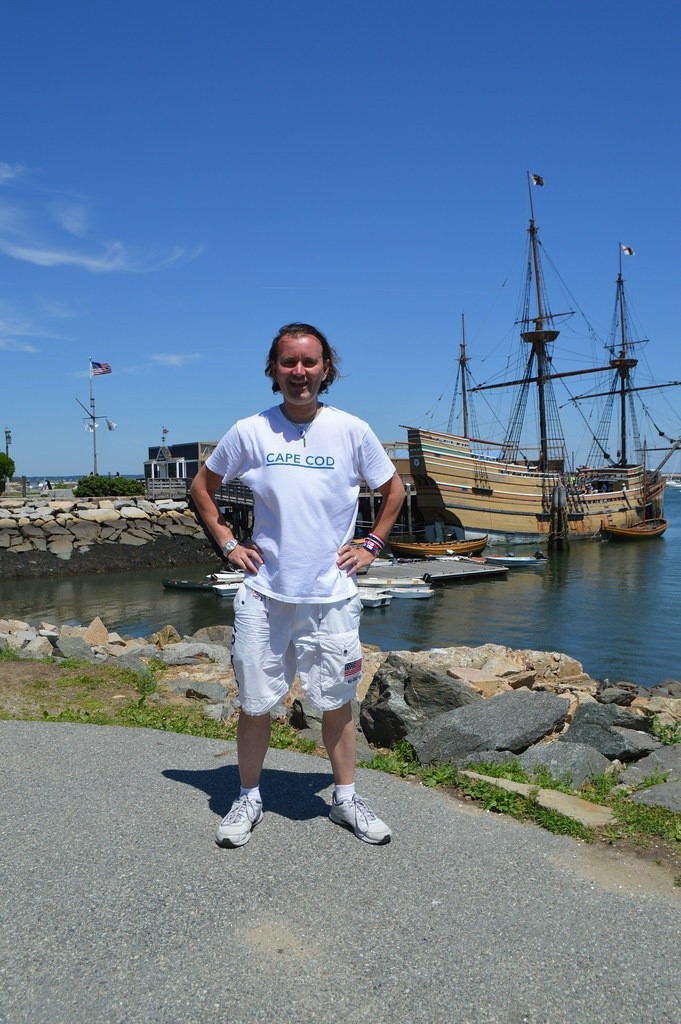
left=215, top=794, right=263, bottom=849
left=329, top=791, right=392, bottom=845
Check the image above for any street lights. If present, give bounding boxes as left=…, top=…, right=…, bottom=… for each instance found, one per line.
left=4, top=426, right=13, bottom=458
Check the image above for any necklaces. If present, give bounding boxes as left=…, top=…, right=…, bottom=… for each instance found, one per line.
left=282, top=403, right=319, bottom=447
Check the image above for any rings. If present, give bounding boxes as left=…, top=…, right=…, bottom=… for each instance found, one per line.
left=354, top=557, right=358, bottom=562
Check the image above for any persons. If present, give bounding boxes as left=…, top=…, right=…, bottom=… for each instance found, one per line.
left=190, top=323, right=405, bottom=848
left=570, top=474, right=606, bottom=494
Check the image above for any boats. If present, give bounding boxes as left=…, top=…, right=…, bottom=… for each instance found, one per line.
left=163, top=556, right=435, bottom=606
left=487, top=549, right=550, bottom=568
left=598, top=436, right=672, bottom=541
left=665, top=478, right=681, bottom=489
left=391, top=531, right=490, bottom=556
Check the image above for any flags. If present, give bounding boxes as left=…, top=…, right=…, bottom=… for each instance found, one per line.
left=92, top=362, right=112, bottom=375
left=86, top=422, right=94, bottom=431
left=622, top=245, right=635, bottom=255
left=531, top=174, right=544, bottom=186
left=106, top=420, right=117, bottom=430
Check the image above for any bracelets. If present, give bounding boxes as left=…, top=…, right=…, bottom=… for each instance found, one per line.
left=362, top=532, right=386, bottom=556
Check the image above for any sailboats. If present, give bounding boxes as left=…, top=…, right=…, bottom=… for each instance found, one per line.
left=395, top=166, right=681, bottom=537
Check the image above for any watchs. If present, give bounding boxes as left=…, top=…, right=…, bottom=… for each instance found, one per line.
left=223, top=538, right=239, bottom=558
